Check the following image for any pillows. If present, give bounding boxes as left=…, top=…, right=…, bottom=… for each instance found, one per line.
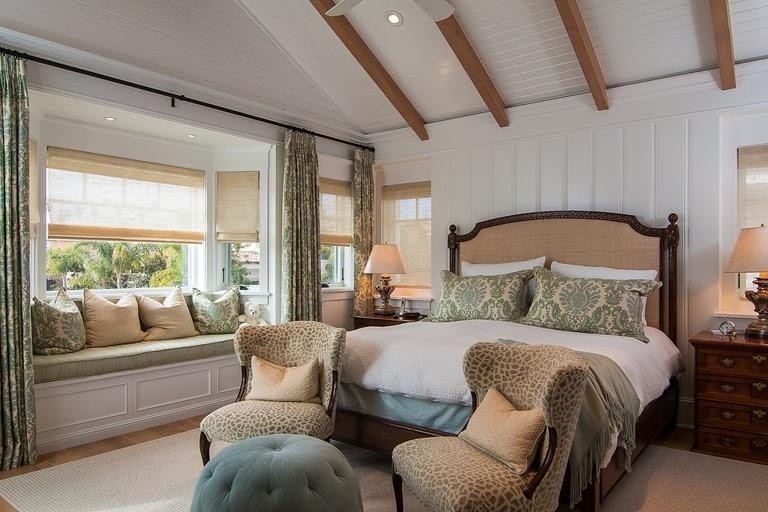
left=550, top=260, right=658, bottom=327
left=245, top=355, right=322, bottom=405
left=518, top=266, right=663, bottom=343
left=458, top=389, right=548, bottom=475
left=421, top=270, right=536, bottom=322
left=32, top=287, right=86, bottom=356
left=82, top=288, right=148, bottom=348
left=135, top=287, right=200, bottom=342
left=461, top=256, right=546, bottom=303
left=191, top=284, right=240, bottom=335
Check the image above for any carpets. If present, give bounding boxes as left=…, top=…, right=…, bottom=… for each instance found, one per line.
left=1, top=431, right=768, bottom=511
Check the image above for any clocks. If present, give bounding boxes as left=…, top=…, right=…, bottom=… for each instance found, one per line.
left=719, top=317, right=734, bottom=335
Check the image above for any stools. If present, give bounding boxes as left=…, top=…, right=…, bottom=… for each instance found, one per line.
left=189, top=433, right=363, bottom=512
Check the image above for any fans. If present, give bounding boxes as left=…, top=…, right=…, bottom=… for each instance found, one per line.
left=324, top=0, right=454, bottom=23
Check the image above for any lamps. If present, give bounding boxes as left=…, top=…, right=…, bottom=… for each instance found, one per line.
left=725, top=223, right=767, bottom=336
left=362, top=242, right=407, bottom=313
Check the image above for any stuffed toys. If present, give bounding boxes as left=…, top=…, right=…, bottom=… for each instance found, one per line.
left=237, top=300, right=270, bottom=329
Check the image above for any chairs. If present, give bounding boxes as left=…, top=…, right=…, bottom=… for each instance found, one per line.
left=198, top=321, right=346, bottom=467
left=390, top=342, right=589, bottom=512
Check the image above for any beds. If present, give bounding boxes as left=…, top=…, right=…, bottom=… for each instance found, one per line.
left=326, top=209, right=679, bottom=512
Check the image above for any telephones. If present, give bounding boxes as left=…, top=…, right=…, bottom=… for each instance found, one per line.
left=399, top=298, right=407, bottom=320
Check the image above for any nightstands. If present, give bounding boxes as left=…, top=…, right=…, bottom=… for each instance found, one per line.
left=352, top=315, right=417, bottom=329
left=688, top=330, right=767, bottom=466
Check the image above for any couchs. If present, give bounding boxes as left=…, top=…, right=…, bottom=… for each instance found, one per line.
left=31, top=294, right=273, bottom=455
